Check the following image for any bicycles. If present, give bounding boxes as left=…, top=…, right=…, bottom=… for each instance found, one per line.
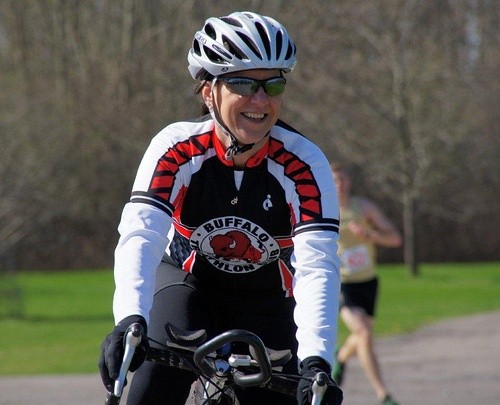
left=102, top=322, right=331, bottom=405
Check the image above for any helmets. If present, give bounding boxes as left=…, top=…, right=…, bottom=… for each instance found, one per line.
left=187, top=11, right=297, bottom=80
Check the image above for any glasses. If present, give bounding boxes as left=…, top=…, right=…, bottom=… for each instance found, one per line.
left=217, top=72, right=288, bottom=96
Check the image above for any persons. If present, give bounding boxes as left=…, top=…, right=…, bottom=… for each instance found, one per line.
left=97, top=11, right=343, bottom=405
left=335, top=162, right=403, bottom=405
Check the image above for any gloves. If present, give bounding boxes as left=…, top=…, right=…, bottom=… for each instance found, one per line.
left=99, top=314, right=149, bottom=393
left=296, top=356, right=344, bottom=405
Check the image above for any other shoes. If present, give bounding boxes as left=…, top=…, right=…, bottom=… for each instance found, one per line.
left=382, top=396, right=397, bottom=405
left=331, top=343, right=345, bottom=387
